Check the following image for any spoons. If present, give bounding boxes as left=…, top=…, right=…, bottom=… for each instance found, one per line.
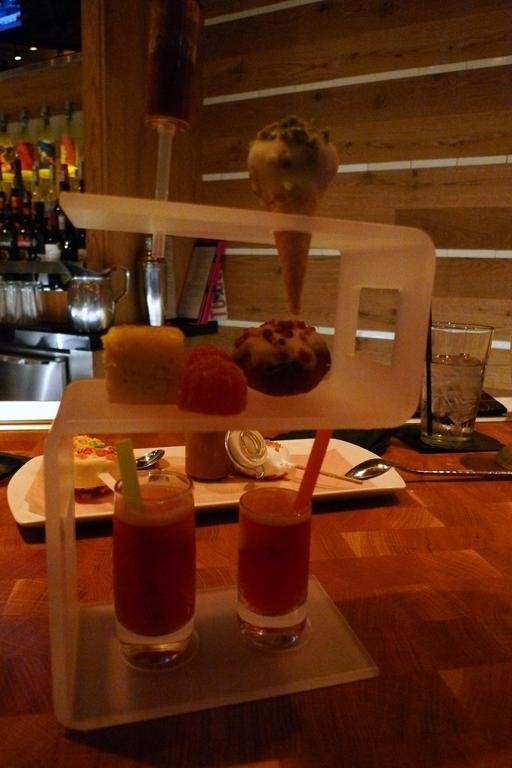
left=133, top=448, right=167, bottom=470
left=346, top=458, right=511, bottom=478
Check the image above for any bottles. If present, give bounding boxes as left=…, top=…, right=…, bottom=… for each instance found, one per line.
left=135, top=236, right=167, bottom=329
left=0, top=276, right=46, bottom=324
left=2, top=104, right=83, bottom=260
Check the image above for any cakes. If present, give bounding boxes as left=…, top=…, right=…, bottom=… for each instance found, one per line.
left=73, top=434, right=121, bottom=502
left=102, top=317, right=331, bottom=416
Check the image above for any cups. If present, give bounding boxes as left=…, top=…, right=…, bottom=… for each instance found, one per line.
left=185, top=433, right=229, bottom=479
left=237, top=487, right=314, bottom=649
left=110, top=472, right=197, bottom=671
left=419, top=319, right=495, bottom=445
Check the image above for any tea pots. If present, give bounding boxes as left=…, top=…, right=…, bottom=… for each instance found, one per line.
left=61, top=253, right=129, bottom=333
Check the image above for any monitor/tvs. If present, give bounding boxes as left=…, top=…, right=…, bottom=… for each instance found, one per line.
left=0, top=0, right=24, bottom=33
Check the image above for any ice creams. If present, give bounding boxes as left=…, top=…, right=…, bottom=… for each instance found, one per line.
left=248, top=115, right=339, bottom=315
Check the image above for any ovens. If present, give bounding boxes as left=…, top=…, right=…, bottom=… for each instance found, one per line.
left=2, top=331, right=102, bottom=402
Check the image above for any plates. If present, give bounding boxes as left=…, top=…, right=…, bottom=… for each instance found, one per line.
left=7, top=437, right=405, bottom=527
